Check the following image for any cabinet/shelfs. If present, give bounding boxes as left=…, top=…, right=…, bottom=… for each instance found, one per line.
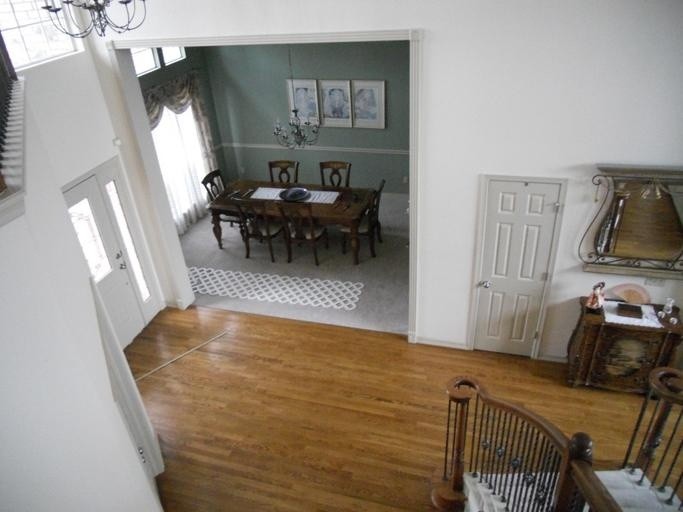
left=565, top=297, right=683, bottom=400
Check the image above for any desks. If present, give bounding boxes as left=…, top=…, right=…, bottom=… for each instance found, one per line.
left=204, top=179, right=376, bottom=265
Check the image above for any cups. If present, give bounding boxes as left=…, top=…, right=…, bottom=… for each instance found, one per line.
left=670, top=317, right=678, bottom=325
left=658, top=311, right=666, bottom=319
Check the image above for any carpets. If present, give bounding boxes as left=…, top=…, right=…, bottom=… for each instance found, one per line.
left=178, top=191, right=409, bottom=334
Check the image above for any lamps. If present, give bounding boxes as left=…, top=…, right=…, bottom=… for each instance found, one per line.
left=41, top=0, right=147, bottom=38
left=272, top=47, right=321, bottom=151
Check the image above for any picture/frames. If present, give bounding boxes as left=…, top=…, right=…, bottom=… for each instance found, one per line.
left=350, top=81, right=386, bottom=130
left=317, top=80, right=353, bottom=128
left=285, top=78, right=320, bottom=128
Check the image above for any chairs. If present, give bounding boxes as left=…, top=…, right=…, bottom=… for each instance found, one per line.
left=335, top=179, right=386, bottom=258
left=276, top=201, right=330, bottom=266
left=201, top=168, right=259, bottom=249
left=269, top=159, right=299, bottom=184
left=319, top=161, right=352, bottom=188
left=233, top=199, right=283, bottom=263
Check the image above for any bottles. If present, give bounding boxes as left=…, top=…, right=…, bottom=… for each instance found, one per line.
left=663, top=297, right=674, bottom=314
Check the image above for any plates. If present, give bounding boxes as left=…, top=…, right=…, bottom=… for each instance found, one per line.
left=279, top=186, right=309, bottom=201
